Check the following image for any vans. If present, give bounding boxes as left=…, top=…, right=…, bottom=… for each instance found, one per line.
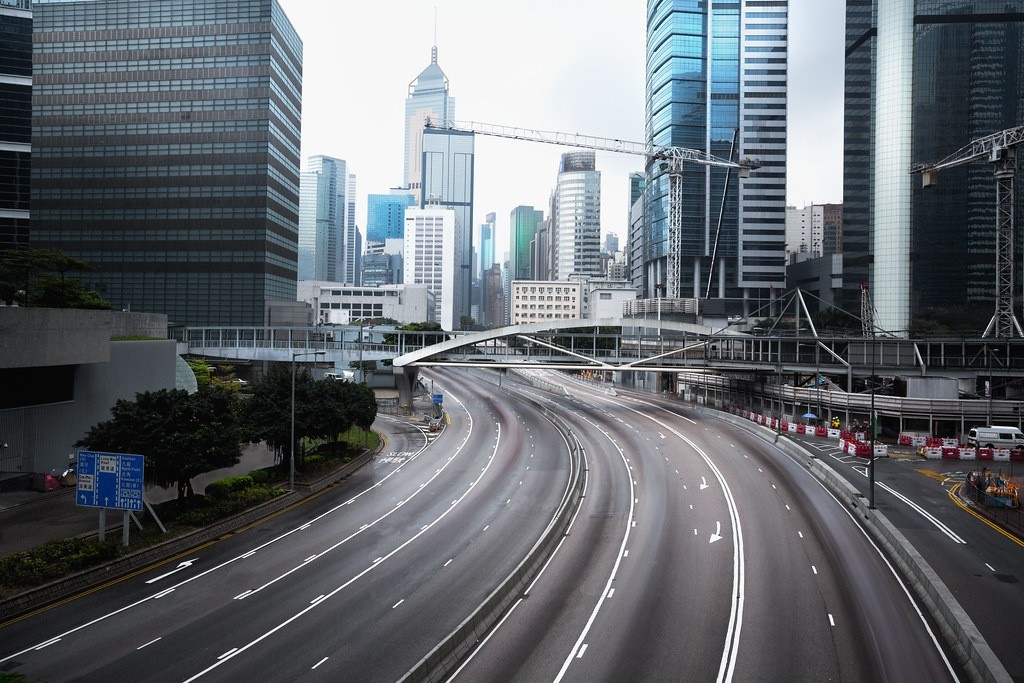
left=968, top=426, right=1024, bottom=450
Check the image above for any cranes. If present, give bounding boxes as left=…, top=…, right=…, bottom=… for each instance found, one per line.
left=908, top=125, right=1024, bottom=338
left=423, top=116, right=742, bottom=298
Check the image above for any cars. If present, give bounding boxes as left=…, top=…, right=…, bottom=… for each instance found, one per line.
left=752, top=327, right=766, bottom=337
left=223, top=378, right=251, bottom=387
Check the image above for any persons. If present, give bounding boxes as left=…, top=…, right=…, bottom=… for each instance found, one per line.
left=831, top=416, right=840, bottom=429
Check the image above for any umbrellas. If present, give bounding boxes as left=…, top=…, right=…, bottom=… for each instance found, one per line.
left=802, top=413, right=819, bottom=425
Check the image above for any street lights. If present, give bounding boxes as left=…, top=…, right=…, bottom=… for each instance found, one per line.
left=290, top=350, right=327, bottom=488
left=860, top=282, right=878, bottom=510
